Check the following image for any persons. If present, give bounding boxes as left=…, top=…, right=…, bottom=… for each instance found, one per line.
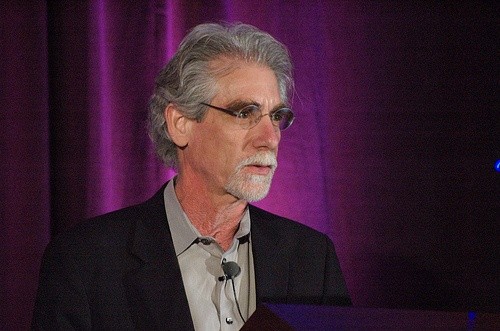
left=31, top=22, right=352, bottom=331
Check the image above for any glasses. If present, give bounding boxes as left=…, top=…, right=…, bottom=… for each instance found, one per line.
left=200, top=102, right=296, bottom=131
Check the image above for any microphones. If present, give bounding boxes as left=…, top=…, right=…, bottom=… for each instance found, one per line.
left=224, top=262, right=241, bottom=278
